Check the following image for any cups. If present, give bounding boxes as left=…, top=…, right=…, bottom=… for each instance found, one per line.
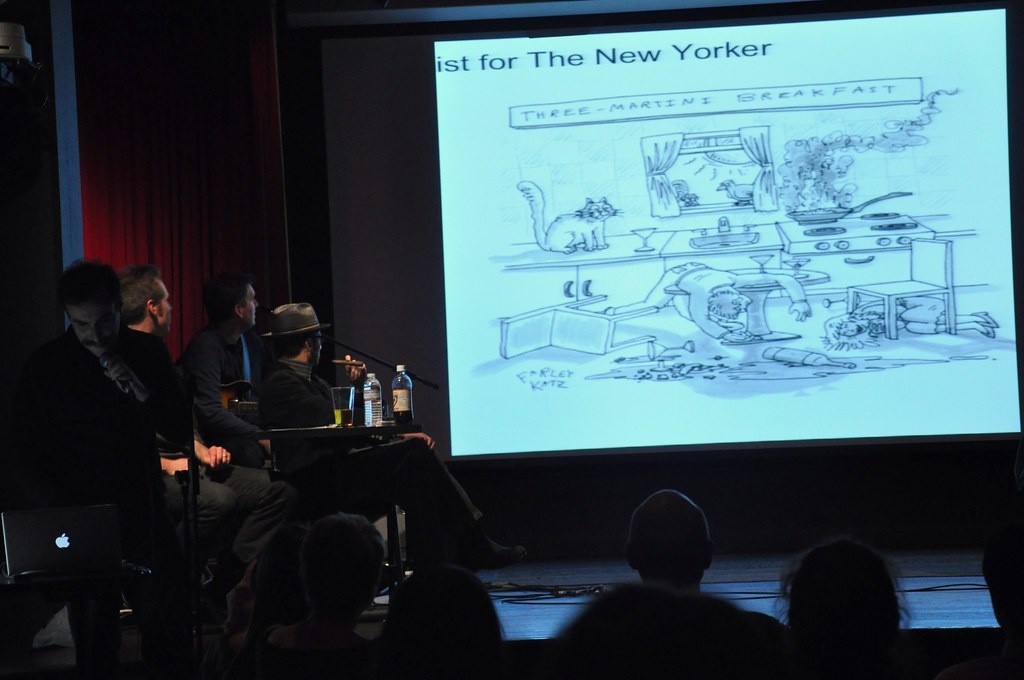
left=331, top=387, right=355, bottom=427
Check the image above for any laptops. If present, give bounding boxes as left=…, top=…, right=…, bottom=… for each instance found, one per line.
left=0, top=503, right=128, bottom=578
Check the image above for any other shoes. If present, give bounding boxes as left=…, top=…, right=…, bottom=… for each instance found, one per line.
left=464, top=524, right=528, bottom=570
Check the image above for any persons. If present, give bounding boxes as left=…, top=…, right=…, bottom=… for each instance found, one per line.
left=202, top=490, right=1024, bottom=680
left=0, top=258, right=525, bottom=679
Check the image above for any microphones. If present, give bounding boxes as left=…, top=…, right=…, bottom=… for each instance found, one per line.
left=98, top=353, right=132, bottom=395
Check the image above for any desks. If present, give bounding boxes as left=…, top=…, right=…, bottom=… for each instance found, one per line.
left=255, top=424, right=422, bottom=580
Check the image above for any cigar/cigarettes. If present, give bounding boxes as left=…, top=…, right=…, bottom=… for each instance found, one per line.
left=332, top=359, right=363, bottom=366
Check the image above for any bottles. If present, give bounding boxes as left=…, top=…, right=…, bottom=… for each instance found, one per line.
left=364, top=373, right=382, bottom=428
left=392, top=365, right=414, bottom=427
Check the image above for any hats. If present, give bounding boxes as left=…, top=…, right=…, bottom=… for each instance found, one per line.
left=260, top=303, right=331, bottom=337
